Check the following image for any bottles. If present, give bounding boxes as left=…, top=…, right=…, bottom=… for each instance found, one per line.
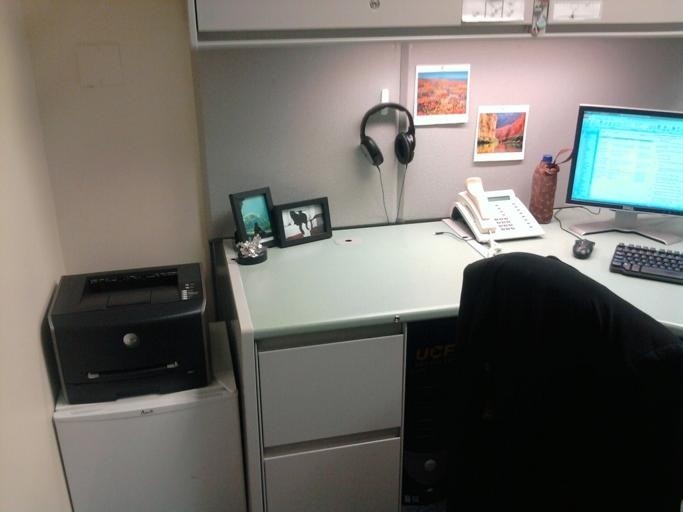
left=529, top=155, right=559, bottom=225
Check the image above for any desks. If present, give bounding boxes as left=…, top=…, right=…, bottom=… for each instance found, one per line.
left=215, top=205, right=683, bottom=512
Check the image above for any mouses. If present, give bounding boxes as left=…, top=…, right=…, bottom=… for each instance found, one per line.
left=572, top=239, right=595, bottom=258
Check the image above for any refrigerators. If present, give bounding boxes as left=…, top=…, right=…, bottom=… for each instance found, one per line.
left=51, top=321, right=247, bottom=512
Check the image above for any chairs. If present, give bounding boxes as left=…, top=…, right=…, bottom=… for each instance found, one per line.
left=450, top=256, right=682, bottom=511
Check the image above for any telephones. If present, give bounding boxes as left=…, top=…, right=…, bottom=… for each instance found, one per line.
left=450, top=176, right=543, bottom=243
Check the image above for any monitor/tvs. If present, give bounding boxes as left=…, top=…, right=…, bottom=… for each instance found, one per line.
left=565, top=104, right=683, bottom=245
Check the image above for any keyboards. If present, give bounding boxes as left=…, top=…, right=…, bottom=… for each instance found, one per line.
left=610, top=243, right=683, bottom=285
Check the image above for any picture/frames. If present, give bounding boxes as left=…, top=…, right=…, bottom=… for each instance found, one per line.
left=229, top=187, right=278, bottom=248
left=273, top=196, right=332, bottom=247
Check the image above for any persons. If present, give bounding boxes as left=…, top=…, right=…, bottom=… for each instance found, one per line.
left=293, top=210, right=310, bottom=236
left=289, top=211, right=305, bottom=236
left=253, top=222, right=265, bottom=239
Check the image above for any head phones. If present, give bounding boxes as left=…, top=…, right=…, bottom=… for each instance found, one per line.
left=360, top=102, right=415, bottom=166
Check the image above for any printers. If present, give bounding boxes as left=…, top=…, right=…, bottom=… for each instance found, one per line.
left=46, top=262, right=213, bottom=403
